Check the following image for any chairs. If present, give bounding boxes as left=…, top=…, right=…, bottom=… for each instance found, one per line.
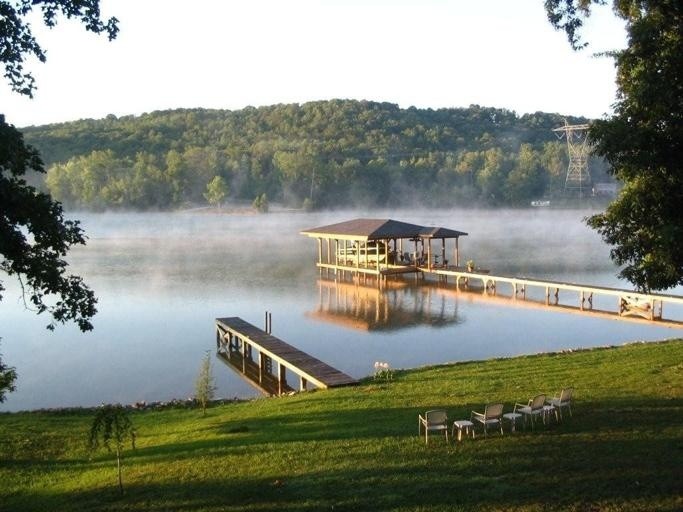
left=414, top=408, right=449, bottom=446
left=467, top=401, right=505, bottom=440
left=513, top=386, right=573, bottom=434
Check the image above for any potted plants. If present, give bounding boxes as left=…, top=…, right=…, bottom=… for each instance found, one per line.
left=466, top=259, right=475, bottom=273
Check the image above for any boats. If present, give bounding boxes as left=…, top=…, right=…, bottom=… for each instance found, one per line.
left=335, top=240, right=398, bottom=266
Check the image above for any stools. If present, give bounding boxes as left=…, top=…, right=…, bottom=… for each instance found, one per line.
left=502, top=412, right=523, bottom=434
left=450, top=420, right=475, bottom=441
